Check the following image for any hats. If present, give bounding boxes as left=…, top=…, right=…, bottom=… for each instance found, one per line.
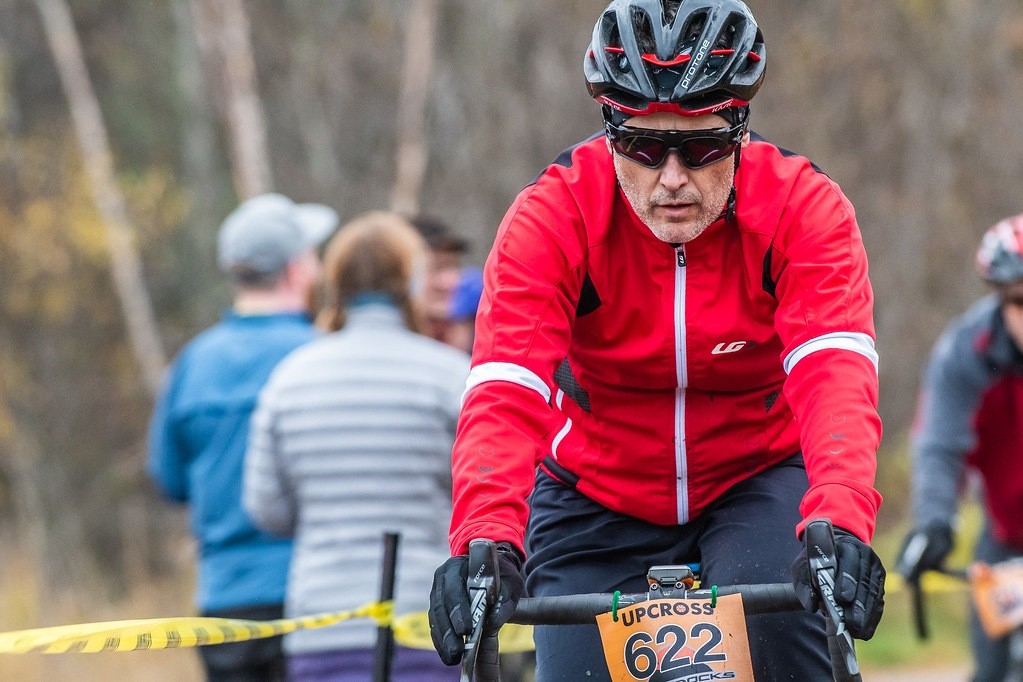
left=602, top=104, right=750, bottom=133
left=214, top=193, right=340, bottom=277
left=444, top=267, right=484, bottom=318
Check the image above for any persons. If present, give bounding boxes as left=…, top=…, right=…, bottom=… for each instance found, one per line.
left=407, top=212, right=484, bottom=354
left=894, top=212, right=1023, bottom=682
left=144, top=195, right=334, bottom=682
left=429, top=0, right=888, bottom=682
left=242, top=209, right=471, bottom=682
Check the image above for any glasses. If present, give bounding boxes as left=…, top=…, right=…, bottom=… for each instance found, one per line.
left=1007, top=294, right=1023, bottom=306
left=598, top=106, right=752, bottom=169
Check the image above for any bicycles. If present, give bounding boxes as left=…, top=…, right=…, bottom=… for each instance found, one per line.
left=906, top=554, right=1023, bottom=682
left=456, top=516, right=866, bottom=682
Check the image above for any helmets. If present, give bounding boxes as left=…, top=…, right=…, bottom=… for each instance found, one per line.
left=975, top=213, right=1023, bottom=284
left=584, top=0, right=768, bottom=102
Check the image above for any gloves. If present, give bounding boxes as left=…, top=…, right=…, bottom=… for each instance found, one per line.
left=428, top=543, right=526, bottom=666
left=792, top=528, right=885, bottom=640
left=896, top=519, right=954, bottom=579
